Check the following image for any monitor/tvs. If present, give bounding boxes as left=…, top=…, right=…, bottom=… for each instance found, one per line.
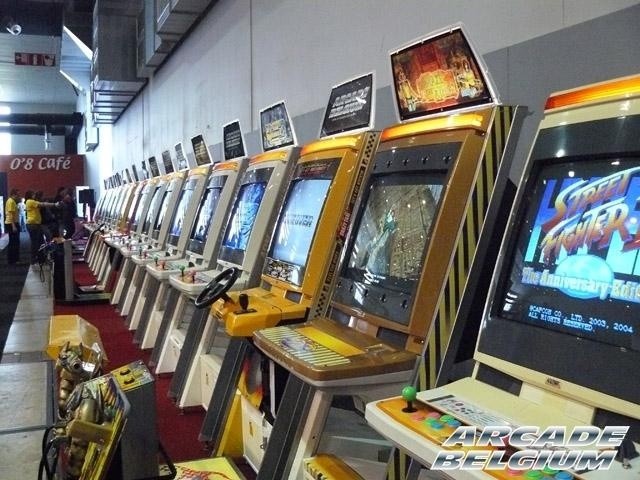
left=154, top=191, right=171, bottom=230
left=169, top=189, right=194, bottom=237
left=222, top=181, right=267, bottom=250
left=339, top=169, right=451, bottom=296
left=189, top=186, right=222, bottom=243
left=267, top=178, right=332, bottom=267
left=490, top=150, right=640, bottom=351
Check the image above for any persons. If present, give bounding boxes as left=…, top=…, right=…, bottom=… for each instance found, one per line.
left=382, top=205, right=398, bottom=232
left=3, top=185, right=75, bottom=266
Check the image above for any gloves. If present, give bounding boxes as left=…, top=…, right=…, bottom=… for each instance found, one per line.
left=54, top=201, right=65, bottom=209
